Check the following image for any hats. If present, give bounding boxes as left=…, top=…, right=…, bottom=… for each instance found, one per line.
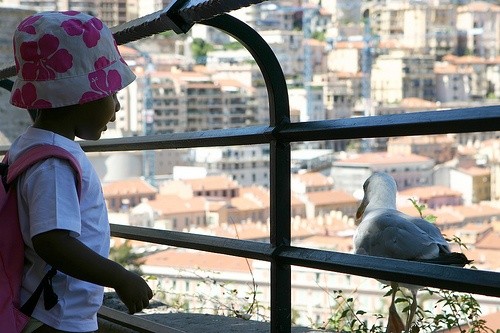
left=9, top=11, right=137, bottom=109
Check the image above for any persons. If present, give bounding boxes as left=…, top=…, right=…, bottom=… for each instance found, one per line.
left=0, top=11, right=153, bottom=332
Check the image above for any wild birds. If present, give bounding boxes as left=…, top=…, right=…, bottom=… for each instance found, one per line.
left=353, top=172, right=475, bottom=333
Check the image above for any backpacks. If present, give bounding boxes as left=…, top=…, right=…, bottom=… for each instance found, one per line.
left=0, top=143, right=82, bottom=333
